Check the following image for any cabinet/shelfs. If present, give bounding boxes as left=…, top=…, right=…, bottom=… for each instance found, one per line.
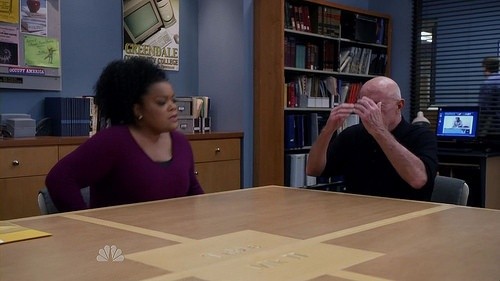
left=1, top=131, right=244, bottom=220
left=254, top=0, right=390, bottom=187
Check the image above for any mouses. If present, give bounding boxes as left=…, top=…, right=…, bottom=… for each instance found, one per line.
left=174, top=34, right=180, bottom=44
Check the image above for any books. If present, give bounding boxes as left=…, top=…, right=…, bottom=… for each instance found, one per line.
left=284, top=35, right=319, bottom=70
left=44, top=96, right=111, bottom=136
left=315, top=5, right=341, bottom=38
left=340, top=47, right=387, bottom=75
left=285, top=75, right=364, bottom=108
left=285, top=153, right=344, bottom=192
left=323, top=40, right=334, bottom=72
left=355, top=14, right=387, bottom=45
left=287, top=113, right=360, bottom=150
left=284, top=2, right=312, bottom=32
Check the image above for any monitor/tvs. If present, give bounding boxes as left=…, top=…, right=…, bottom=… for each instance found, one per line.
left=435, top=105, right=480, bottom=139
left=123, top=1, right=164, bottom=43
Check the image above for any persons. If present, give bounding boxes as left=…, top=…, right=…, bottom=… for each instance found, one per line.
left=478, top=57, right=500, bottom=133
left=44, top=56, right=204, bottom=211
left=305, top=76, right=438, bottom=202
left=453, top=117, right=463, bottom=128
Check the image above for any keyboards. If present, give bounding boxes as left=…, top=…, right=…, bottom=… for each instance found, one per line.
left=142, top=31, right=172, bottom=49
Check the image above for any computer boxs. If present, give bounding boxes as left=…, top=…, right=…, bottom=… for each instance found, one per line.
left=154, top=0, right=177, bottom=28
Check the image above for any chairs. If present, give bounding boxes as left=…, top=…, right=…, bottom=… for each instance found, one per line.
left=427, top=175, right=468, bottom=207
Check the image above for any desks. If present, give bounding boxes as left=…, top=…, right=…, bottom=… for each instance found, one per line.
left=0, top=184, right=500, bottom=281
left=436, top=141, right=500, bottom=208
